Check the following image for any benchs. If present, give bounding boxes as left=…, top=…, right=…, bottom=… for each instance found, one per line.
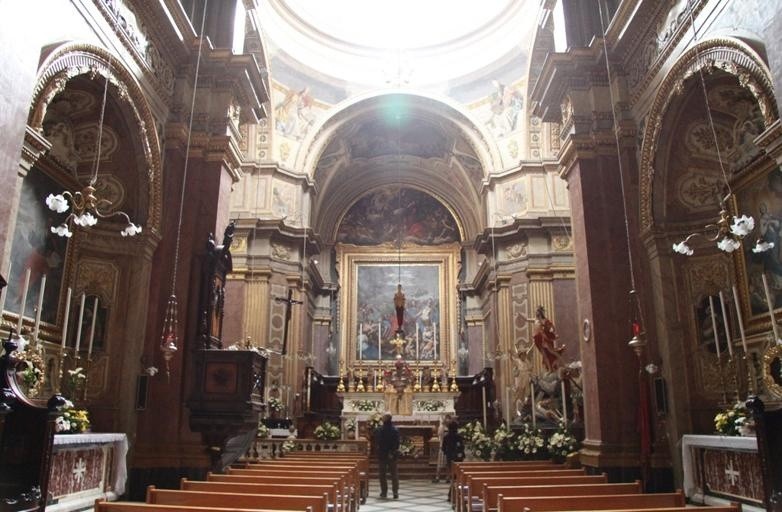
left=446, top=456, right=743, bottom=511
left=95, top=446, right=368, bottom=512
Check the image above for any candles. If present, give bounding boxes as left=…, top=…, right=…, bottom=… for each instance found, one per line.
left=530, top=381, right=537, bottom=426
left=561, top=380, right=568, bottom=427
left=505, top=386, right=510, bottom=433
left=708, top=285, right=748, bottom=357
left=378, top=322, right=382, bottom=360
left=482, top=385, right=488, bottom=428
left=433, top=322, right=437, bottom=359
left=415, top=322, right=419, bottom=359
left=1, top=259, right=98, bottom=363
left=360, top=322, right=363, bottom=360
left=760, top=271, right=780, bottom=343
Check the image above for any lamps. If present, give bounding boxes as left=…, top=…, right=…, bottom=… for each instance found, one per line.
left=44, top=1, right=144, bottom=239
left=160, top=2, right=211, bottom=378
left=592, top=1, right=649, bottom=360
left=668, top=1, right=777, bottom=256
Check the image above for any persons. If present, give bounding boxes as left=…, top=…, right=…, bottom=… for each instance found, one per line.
left=335, top=185, right=454, bottom=245
left=514, top=392, right=563, bottom=425
left=748, top=275, right=776, bottom=312
left=392, top=354, right=412, bottom=379
left=456, top=340, right=471, bottom=377
left=393, top=283, right=406, bottom=329
left=486, top=76, right=522, bottom=159
left=753, top=198, right=781, bottom=279
left=356, top=288, right=441, bottom=362
left=78, top=305, right=106, bottom=351
left=374, top=413, right=402, bottom=498
left=222, top=217, right=236, bottom=256
left=441, top=421, right=466, bottom=474
left=513, top=304, right=567, bottom=377
left=275, top=82, right=316, bottom=136
left=15, top=211, right=60, bottom=317
left=511, top=340, right=539, bottom=415
left=697, top=303, right=726, bottom=356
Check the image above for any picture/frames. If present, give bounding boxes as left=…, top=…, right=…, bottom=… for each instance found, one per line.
left=332, top=238, right=460, bottom=379
left=0, top=154, right=83, bottom=358
left=724, top=150, right=782, bottom=346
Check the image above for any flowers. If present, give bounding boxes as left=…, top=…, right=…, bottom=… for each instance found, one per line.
left=16, top=359, right=43, bottom=396
left=457, top=416, right=579, bottom=458
left=68, top=366, right=88, bottom=400
left=257, top=397, right=341, bottom=455
left=51, top=399, right=90, bottom=434
left=712, top=396, right=758, bottom=437
